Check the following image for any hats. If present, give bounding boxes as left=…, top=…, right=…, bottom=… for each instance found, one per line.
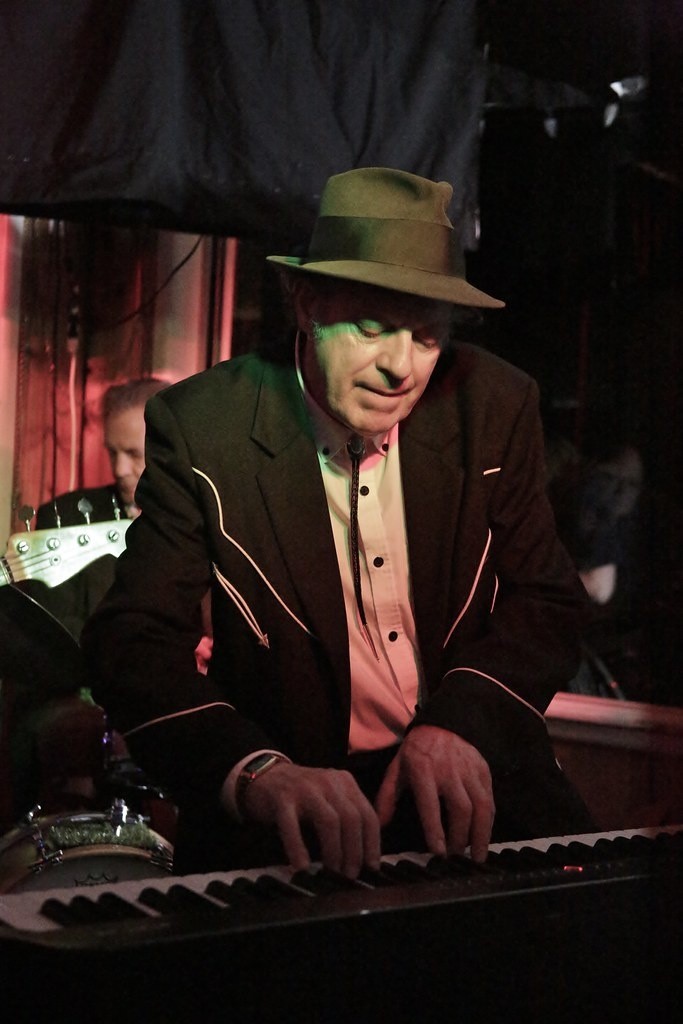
left=266, top=167, right=506, bottom=309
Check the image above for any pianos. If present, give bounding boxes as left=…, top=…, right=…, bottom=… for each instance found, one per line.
left=0, top=820, right=683, bottom=1015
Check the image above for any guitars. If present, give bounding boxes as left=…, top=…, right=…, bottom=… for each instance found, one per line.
left=1, top=496, right=137, bottom=594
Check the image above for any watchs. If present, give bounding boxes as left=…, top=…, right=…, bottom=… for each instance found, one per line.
left=236, top=753, right=290, bottom=824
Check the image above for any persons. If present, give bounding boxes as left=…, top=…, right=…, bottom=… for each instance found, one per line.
left=81, top=168, right=595, bottom=879
left=29, top=377, right=666, bottom=703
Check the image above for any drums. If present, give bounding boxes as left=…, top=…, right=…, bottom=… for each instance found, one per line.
left=0, top=806, right=185, bottom=897
